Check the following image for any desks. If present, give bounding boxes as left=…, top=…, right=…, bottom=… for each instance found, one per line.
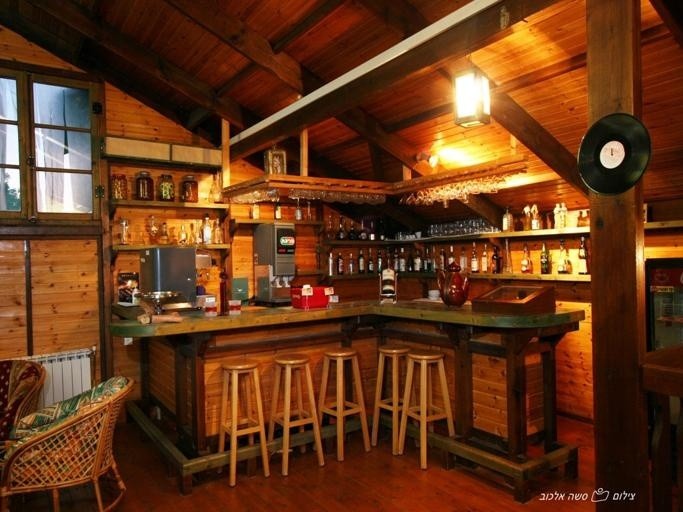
left=111, top=297, right=586, bottom=503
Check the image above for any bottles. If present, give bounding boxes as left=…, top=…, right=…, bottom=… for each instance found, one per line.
left=553, top=235, right=573, bottom=275
left=293, top=200, right=304, bottom=221
left=211, top=217, right=224, bottom=245
left=492, top=245, right=503, bottom=275
left=551, top=202, right=562, bottom=229
left=109, top=172, right=130, bottom=202
left=459, top=243, right=470, bottom=273
left=156, top=172, right=178, bottom=202
left=376, top=249, right=384, bottom=275
left=560, top=201, right=571, bottom=228
left=574, top=206, right=592, bottom=228
left=187, top=222, right=201, bottom=248
left=358, top=217, right=369, bottom=240
left=423, top=246, right=434, bottom=274
left=367, top=246, right=375, bottom=273
left=530, top=202, right=542, bottom=230
left=273, top=200, right=283, bottom=221
left=337, top=252, right=346, bottom=275
left=521, top=201, right=531, bottom=230
left=479, top=242, right=490, bottom=274
left=368, top=217, right=377, bottom=240
left=446, top=244, right=462, bottom=273
left=116, top=216, right=133, bottom=248
left=412, top=244, right=423, bottom=272
left=357, top=249, right=366, bottom=275
left=469, top=241, right=481, bottom=274
left=206, top=173, right=224, bottom=204
left=251, top=202, right=262, bottom=222
left=438, top=245, right=447, bottom=273
left=539, top=239, right=551, bottom=275
left=502, top=203, right=515, bottom=232
left=134, top=169, right=155, bottom=201
left=398, top=247, right=408, bottom=273
left=199, top=212, right=214, bottom=245
left=577, top=236, right=589, bottom=274
left=348, top=252, right=357, bottom=277
left=392, top=247, right=400, bottom=272
left=520, top=241, right=534, bottom=274
left=324, top=210, right=337, bottom=241
left=327, top=250, right=335, bottom=277
left=159, top=222, right=169, bottom=244
left=337, top=214, right=348, bottom=241
left=348, top=219, right=359, bottom=241
left=181, top=173, right=199, bottom=203
left=177, top=223, right=189, bottom=248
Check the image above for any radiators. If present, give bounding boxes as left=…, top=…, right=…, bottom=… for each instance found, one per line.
left=1, top=343, right=96, bottom=410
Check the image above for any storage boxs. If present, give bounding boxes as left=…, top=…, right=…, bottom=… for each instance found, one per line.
left=291, top=287, right=330, bottom=309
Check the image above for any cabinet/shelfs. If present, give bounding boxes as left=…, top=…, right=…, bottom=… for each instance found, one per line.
left=105, top=135, right=228, bottom=312
left=231, top=198, right=325, bottom=244
left=319, top=196, right=591, bottom=284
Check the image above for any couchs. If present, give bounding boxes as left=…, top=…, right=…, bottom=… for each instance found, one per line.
left=0, top=358, right=46, bottom=448
left=0, top=376, right=136, bottom=512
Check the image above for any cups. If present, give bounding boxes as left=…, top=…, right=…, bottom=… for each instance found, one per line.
left=428, top=289, right=440, bottom=301
left=204, top=301, right=217, bottom=317
left=228, top=299, right=242, bottom=316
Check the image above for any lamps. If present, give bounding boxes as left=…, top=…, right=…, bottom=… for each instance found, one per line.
left=453, top=55, right=491, bottom=129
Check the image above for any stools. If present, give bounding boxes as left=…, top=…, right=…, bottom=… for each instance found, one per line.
left=317, top=348, right=371, bottom=461
left=268, top=354, right=324, bottom=475
left=399, top=350, right=455, bottom=469
left=371, top=345, right=420, bottom=455
left=215, top=360, right=269, bottom=487
